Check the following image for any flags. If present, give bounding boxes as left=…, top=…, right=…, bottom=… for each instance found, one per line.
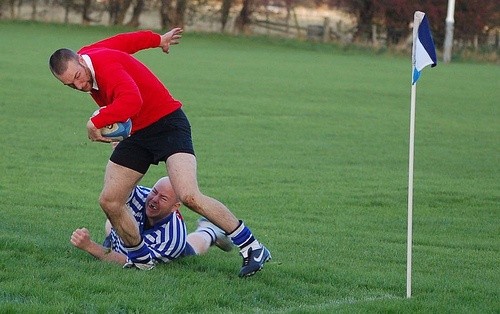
left=412, top=13, right=437, bottom=85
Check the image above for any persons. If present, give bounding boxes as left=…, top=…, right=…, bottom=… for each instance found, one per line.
left=71, top=142, right=234, bottom=269
left=49, top=27, right=271, bottom=278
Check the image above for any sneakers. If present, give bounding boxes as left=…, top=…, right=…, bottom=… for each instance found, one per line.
left=201, top=217, right=235, bottom=252
left=239, top=246, right=273, bottom=278
left=121, top=255, right=160, bottom=271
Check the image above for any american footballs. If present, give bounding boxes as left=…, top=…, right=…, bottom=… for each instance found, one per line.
left=92, top=106, right=132, bottom=143
